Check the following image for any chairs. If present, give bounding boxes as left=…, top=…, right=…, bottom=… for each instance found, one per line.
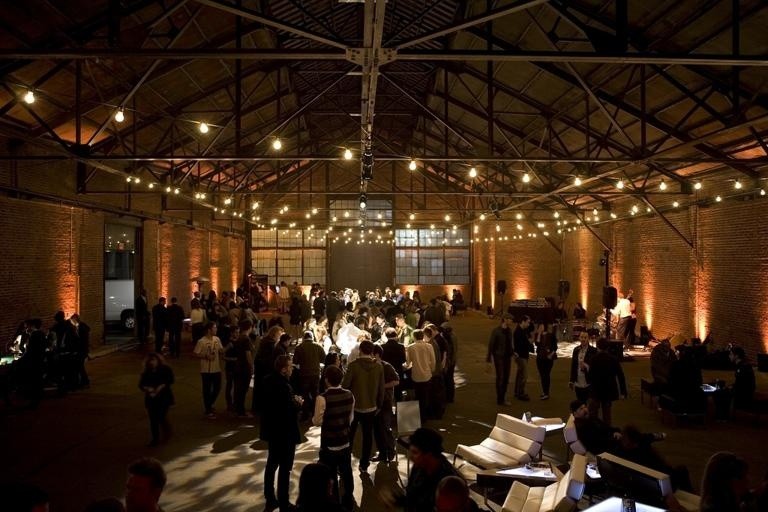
left=596, top=451, right=704, bottom=512
left=501, top=454, right=587, bottom=512
left=392, top=398, right=426, bottom=491
left=453, top=411, right=547, bottom=474
left=562, top=413, right=598, bottom=468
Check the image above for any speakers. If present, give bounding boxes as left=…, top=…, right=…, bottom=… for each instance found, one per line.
left=497, top=280, right=507, bottom=295
left=558, top=281, right=569, bottom=299
left=602, top=285, right=618, bottom=310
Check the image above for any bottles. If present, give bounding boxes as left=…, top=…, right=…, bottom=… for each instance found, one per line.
left=579, top=357, right=584, bottom=370
left=623, top=482, right=635, bottom=512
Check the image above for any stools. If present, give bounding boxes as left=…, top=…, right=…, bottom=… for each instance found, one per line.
left=641, top=377, right=659, bottom=405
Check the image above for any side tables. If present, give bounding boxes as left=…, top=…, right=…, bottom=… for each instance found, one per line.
left=535, top=419, right=567, bottom=462
left=477, top=463, right=566, bottom=504
left=580, top=495, right=666, bottom=512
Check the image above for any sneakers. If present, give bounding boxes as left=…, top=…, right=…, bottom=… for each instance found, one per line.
left=514, top=393, right=530, bottom=400
left=497, top=399, right=513, bottom=405
left=541, top=395, right=550, bottom=400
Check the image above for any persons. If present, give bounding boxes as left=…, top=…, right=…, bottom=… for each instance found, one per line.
left=10, top=312, right=90, bottom=400
left=572, top=302, right=585, bottom=321
left=122, top=459, right=165, bottom=512
left=152, top=297, right=168, bottom=353
left=562, top=333, right=756, bottom=512
left=617, top=293, right=637, bottom=347
left=167, top=296, right=184, bottom=356
left=84, top=496, right=124, bottom=510
left=486, top=313, right=557, bottom=406
left=555, top=302, right=567, bottom=320
left=11, top=484, right=49, bottom=511
left=190, top=279, right=468, bottom=511
left=139, top=351, right=174, bottom=447
left=135, top=288, right=150, bottom=342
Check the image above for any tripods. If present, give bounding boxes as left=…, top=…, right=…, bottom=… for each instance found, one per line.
left=492, top=297, right=509, bottom=324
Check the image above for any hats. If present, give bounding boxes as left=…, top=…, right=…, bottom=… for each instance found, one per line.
left=303, top=331, right=315, bottom=341
left=501, top=312, right=514, bottom=319
left=410, top=427, right=445, bottom=452
left=570, top=400, right=587, bottom=413
